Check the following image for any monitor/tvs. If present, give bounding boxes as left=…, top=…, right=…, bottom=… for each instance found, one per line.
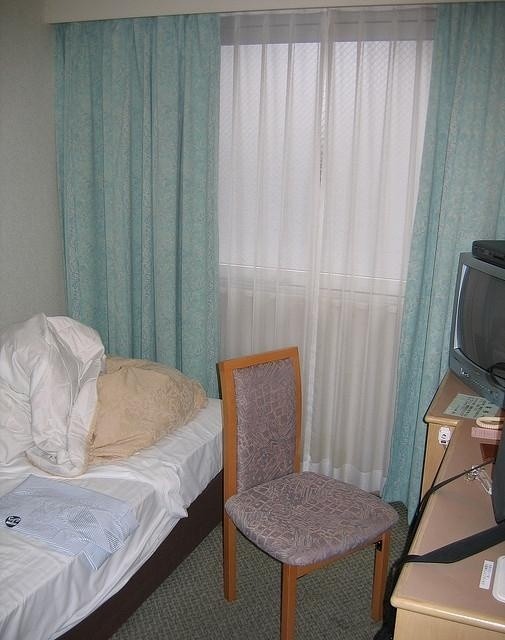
left=448, top=252, right=505, bottom=410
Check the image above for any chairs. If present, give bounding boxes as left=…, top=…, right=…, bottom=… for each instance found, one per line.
left=216, top=347, right=398, bottom=640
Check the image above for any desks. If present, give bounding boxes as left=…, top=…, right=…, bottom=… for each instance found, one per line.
left=390, top=422, right=504, bottom=640
left=420, top=368, right=504, bottom=502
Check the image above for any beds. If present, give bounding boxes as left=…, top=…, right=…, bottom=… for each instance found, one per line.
left=0, top=315, right=222, bottom=640
